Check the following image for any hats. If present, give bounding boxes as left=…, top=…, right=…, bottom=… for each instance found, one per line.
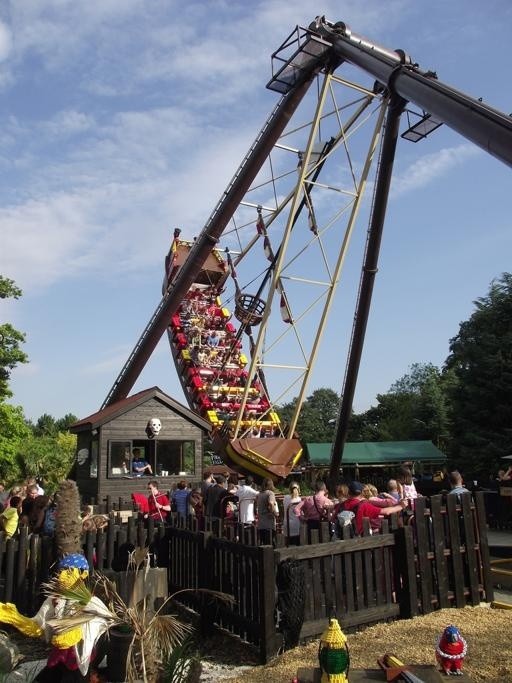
left=351, top=481, right=361, bottom=493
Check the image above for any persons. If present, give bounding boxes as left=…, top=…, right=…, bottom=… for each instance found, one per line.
left=0, top=554, right=116, bottom=683
left=163, top=281, right=285, bottom=440
left=149, top=418, right=162, bottom=438
left=131, top=448, right=153, bottom=476
